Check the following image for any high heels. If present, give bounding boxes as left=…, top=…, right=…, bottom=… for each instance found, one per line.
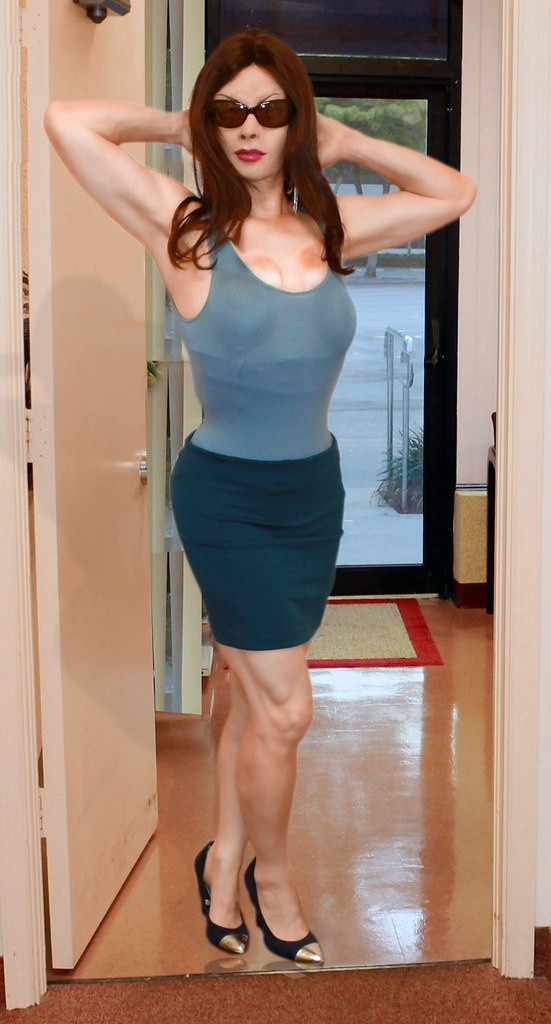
left=245, top=856, right=324, bottom=965
left=195, top=841, right=249, bottom=954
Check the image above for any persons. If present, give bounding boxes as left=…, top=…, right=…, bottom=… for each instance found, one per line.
left=42, top=32, right=477, bottom=963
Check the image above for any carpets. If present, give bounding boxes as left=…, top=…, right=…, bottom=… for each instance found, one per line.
left=307, top=598, right=445, bottom=668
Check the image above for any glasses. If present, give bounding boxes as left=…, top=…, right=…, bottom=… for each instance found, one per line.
left=213, top=99, right=294, bottom=128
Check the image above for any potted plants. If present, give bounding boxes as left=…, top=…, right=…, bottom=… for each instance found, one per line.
left=370, top=423, right=423, bottom=514
